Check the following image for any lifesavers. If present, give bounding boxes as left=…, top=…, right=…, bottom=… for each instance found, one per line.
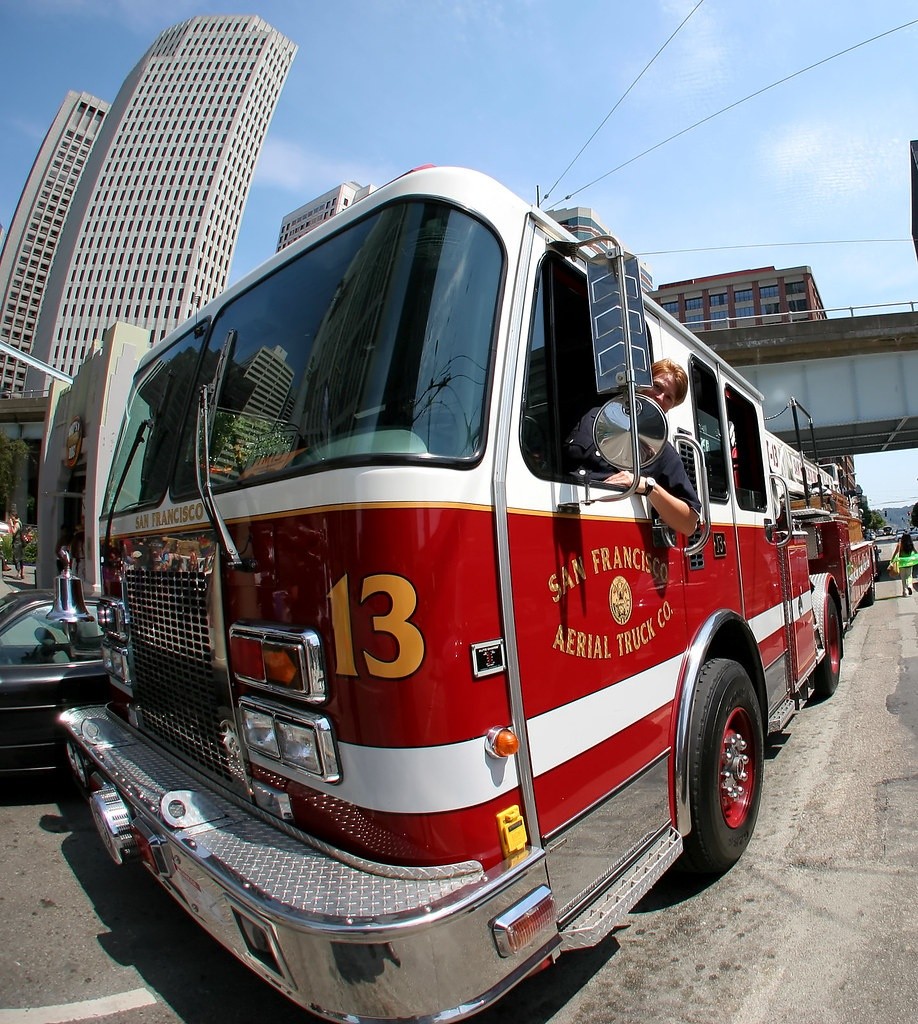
left=891, top=553, right=918, bottom=568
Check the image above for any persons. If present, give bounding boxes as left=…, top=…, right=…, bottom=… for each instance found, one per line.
left=891, top=534, right=918, bottom=596
left=0, top=510, right=34, bottom=580
left=102, top=533, right=216, bottom=583
left=561, top=356, right=702, bottom=538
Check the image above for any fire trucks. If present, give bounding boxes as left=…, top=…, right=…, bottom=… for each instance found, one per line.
left=49, top=161, right=881, bottom=1024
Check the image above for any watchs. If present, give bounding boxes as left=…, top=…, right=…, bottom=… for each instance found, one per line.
left=641, top=476, right=655, bottom=496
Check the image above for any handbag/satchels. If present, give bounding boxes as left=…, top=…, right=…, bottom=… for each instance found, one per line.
left=888, top=561, right=899, bottom=577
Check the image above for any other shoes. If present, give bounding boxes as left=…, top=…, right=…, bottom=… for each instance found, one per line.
left=903, top=592, right=908, bottom=596
left=907, top=586, right=913, bottom=595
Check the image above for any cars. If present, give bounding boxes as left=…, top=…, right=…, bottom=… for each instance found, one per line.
left=883, top=525, right=918, bottom=542
left=0, top=589, right=109, bottom=779
